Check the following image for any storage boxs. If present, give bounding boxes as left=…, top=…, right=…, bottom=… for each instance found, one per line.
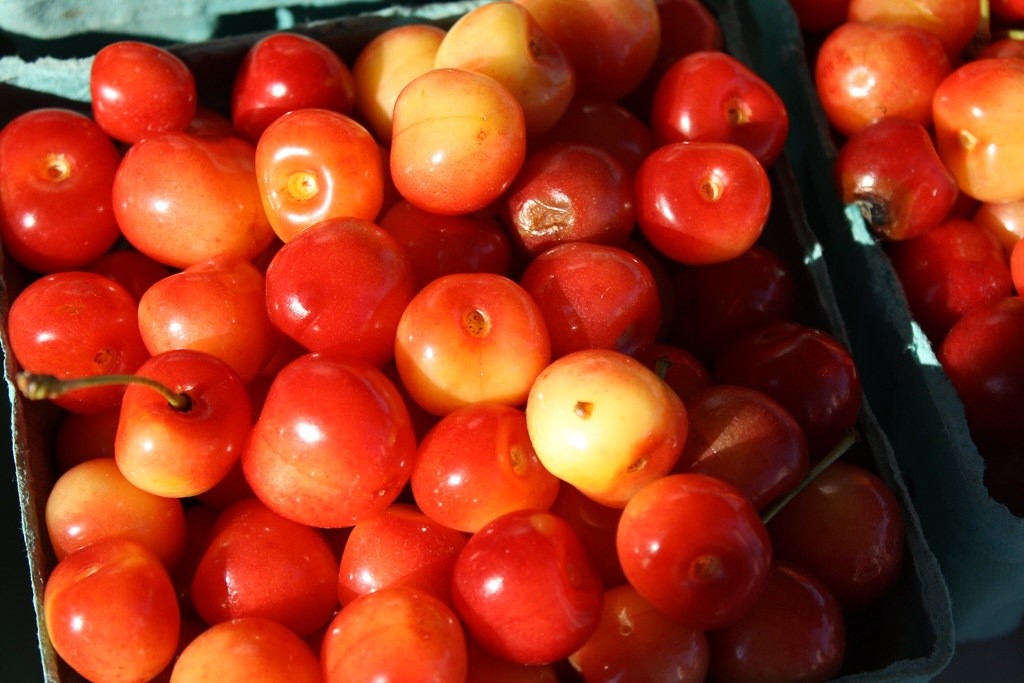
left=0, top=1, right=1024, bottom=683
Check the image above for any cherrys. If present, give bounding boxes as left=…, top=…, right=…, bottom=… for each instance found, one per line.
left=0, top=0, right=1024, bottom=683
